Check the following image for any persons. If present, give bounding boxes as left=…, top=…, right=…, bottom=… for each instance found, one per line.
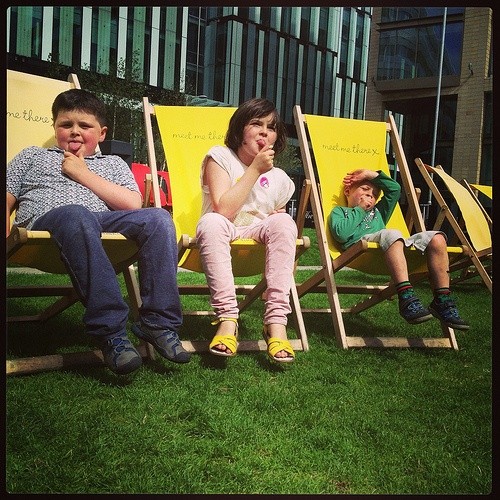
left=329, top=170, right=469, bottom=330
left=7, top=89, right=190, bottom=375
left=196, top=99, right=299, bottom=360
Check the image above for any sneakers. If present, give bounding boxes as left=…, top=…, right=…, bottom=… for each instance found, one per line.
left=399, top=294, right=432, bottom=324
left=428, top=297, right=470, bottom=331
left=130, top=318, right=191, bottom=363
left=97, top=332, right=142, bottom=374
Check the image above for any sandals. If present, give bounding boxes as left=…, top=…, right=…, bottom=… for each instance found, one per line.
left=261, top=324, right=295, bottom=362
left=208, top=317, right=239, bottom=357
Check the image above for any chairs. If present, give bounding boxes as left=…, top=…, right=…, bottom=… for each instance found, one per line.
left=7, top=73, right=493, bottom=374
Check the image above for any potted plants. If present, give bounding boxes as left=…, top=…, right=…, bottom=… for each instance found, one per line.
left=75, top=51, right=145, bottom=170
